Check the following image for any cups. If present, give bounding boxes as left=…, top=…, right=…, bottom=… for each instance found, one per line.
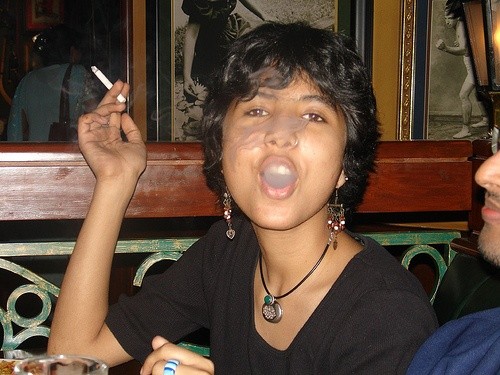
left=14, top=354, right=109, bottom=375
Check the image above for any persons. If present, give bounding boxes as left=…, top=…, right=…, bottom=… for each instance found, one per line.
left=47, top=20, right=439, bottom=375
left=181, top=0, right=253, bottom=99
left=140, top=123, right=499, bottom=375
left=436, top=7, right=488, bottom=138
left=1, top=23, right=172, bottom=142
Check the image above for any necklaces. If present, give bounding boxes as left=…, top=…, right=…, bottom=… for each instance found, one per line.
left=259, top=230, right=334, bottom=325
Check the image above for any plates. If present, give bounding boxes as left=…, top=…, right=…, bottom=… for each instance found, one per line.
left=0, top=358, right=15, bottom=366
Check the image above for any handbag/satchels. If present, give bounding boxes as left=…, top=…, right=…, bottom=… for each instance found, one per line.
left=48, top=63, right=78, bottom=141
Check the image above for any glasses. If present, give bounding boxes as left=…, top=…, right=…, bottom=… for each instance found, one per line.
left=489, top=127, right=499, bottom=155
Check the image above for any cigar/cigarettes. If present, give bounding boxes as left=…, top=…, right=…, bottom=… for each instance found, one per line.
left=91, top=65, right=125, bottom=103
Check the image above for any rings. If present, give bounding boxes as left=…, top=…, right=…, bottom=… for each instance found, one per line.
left=164, top=359, right=178, bottom=374
